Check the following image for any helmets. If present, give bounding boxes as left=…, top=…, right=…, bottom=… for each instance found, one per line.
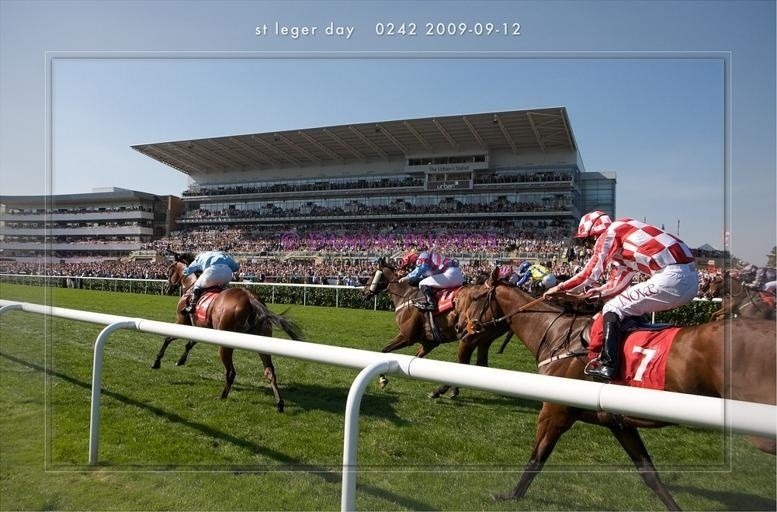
left=519, top=261, right=531, bottom=274
left=574, top=211, right=613, bottom=239
left=401, top=253, right=419, bottom=268
left=743, top=265, right=758, bottom=276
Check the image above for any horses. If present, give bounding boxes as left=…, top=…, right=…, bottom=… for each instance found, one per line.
left=496, top=267, right=596, bottom=354
left=454, top=266, right=777, bottom=512
left=362, top=256, right=509, bottom=399
left=151, top=253, right=304, bottom=412
left=707, top=270, right=776, bottom=324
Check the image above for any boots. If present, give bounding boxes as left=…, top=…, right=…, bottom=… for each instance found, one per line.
left=185, top=287, right=203, bottom=314
left=586, top=311, right=623, bottom=382
left=418, top=284, right=437, bottom=311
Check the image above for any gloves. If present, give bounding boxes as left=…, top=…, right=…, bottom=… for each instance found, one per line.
left=741, top=281, right=749, bottom=287
left=398, top=276, right=411, bottom=285
left=543, top=285, right=565, bottom=302
